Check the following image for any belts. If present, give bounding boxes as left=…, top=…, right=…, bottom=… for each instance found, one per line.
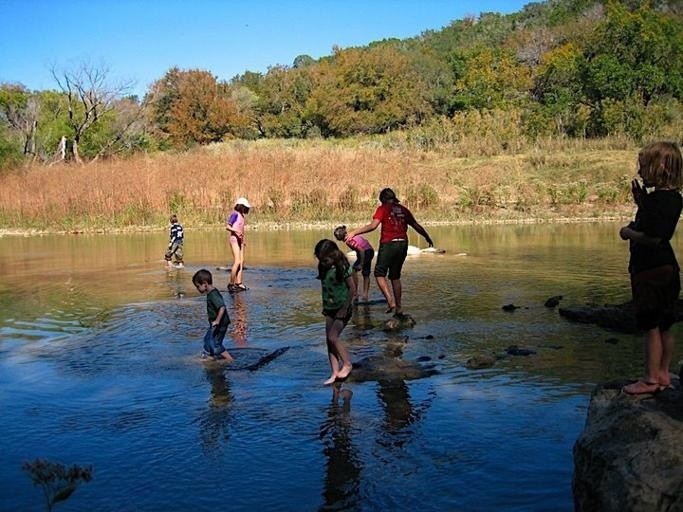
left=391, top=238, right=405, bottom=242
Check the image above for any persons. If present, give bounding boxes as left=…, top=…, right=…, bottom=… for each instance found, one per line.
left=343, top=187, right=434, bottom=317
left=191, top=268, right=233, bottom=362
left=333, top=226, right=374, bottom=302
left=312, top=238, right=357, bottom=385
left=618, top=141, right=682, bottom=395
left=226, top=196, right=251, bottom=292
left=164, top=214, right=185, bottom=266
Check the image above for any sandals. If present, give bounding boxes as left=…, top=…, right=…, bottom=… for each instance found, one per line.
left=235, top=282, right=249, bottom=291
left=226, top=283, right=238, bottom=292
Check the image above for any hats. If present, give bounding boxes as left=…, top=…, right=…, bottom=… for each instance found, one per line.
left=236, top=198, right=250, bottom=208
